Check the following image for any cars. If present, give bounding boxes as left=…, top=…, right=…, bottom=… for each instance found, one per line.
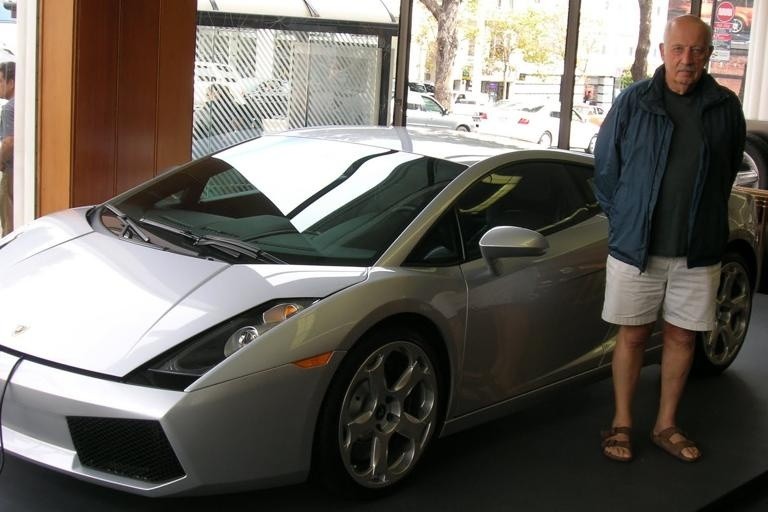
left=474, top=97, right=601, bottom=152
left=389, top=79, right=507, bottom=132
left=244, top=79, right=290, bottom=120
left=1, top=124, right=762, bottom=503
left=194, top=64, right=247, bottom=109
left=574, top=103, right=607, bottom=125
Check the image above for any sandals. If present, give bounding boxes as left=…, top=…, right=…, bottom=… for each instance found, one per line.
left=599, top=423, right=633, bottom=464
left=646, top=423, right=705, bottom=464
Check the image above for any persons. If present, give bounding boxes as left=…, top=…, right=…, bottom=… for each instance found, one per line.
left=0, top=61, right=16, bottom=239
left=588, top=13, right=746, bottom=461
